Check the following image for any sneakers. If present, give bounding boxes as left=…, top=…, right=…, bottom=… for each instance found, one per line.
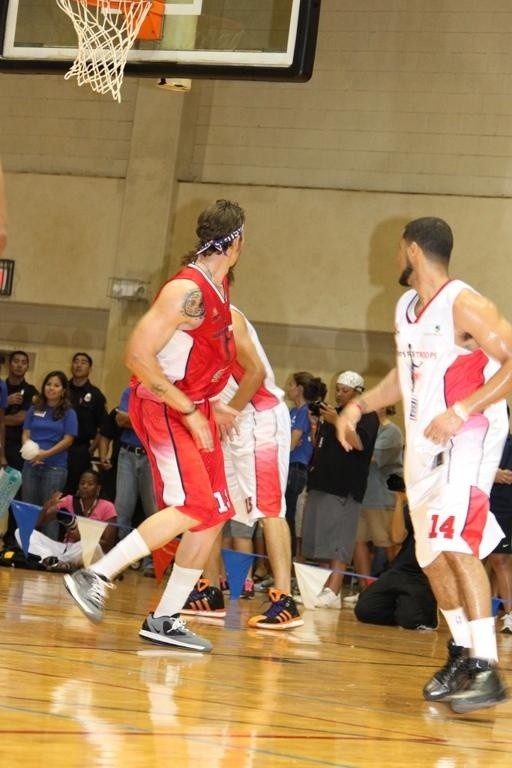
left=24, top=535, right=511, bottom=714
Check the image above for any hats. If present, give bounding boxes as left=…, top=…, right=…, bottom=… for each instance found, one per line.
left=329, top=368, right=360, bottom=394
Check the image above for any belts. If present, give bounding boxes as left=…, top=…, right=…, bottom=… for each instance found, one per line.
left=122, top=442, right=152, bottom=456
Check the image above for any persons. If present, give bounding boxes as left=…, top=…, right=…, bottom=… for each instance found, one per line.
left=333, top=216, right=512, bottom=715
left=1, top=351, right=512, bottom=634
left=178, top=245, right=304, bottom=629
left=62, top=198, right=246, bottom=653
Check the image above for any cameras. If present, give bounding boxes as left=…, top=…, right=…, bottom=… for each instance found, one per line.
left=57, top=507, right=77, bottom=529
left=308, top=402, right=323, bottom=416
